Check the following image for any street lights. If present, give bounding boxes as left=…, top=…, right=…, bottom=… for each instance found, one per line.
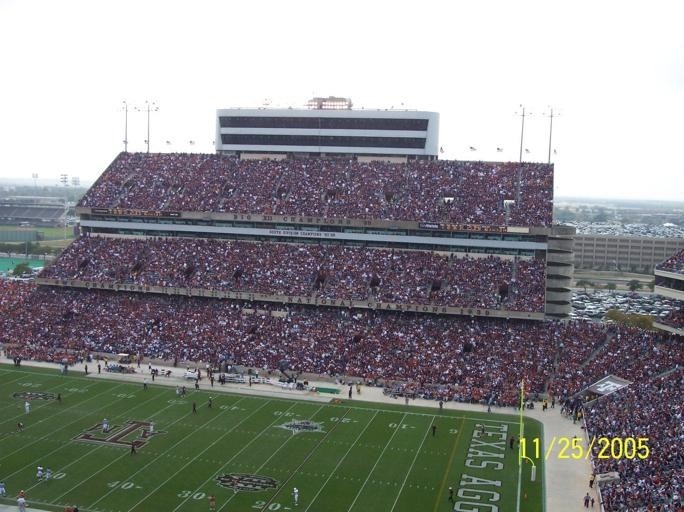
left=515, top=103, right=533, bottom=162
left=32, top=172, right=38, bottom=196
left=134, top=100, right=159, bottom=154
left=542, top=104, right=560, bottom=163
left=122, top=100, right=128, bottom=151
left=60, top=174, right=68, bottom=241
left=72, top=177, right=79, bottom=202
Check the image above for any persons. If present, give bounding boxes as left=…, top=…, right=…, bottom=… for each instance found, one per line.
left=100, top=417, right=110, bottom=432
left=509, top=436, right=514, bottom=450
left=208, top=494, right=217, bottom=512
left=291, top=487, right=299, bottom=506
left=431, top=423, right=436, bottom=436
left=19, top=421, right=24, bottom=433
left=1, top=278, right=573, bottom=417
left=62, top=505, right=73, bottom=512
left=44, top=466, right=53, bottom=481
left=0, top=480, right=6, bottom=497
left=447, top=486, right=456, bottom=504
left=573, top=246, right=683, bottom=512
left=149, top=422, right=153, bottom=435
left=71, top=505, right=79, bottom=512
left=479, top=423, right=487, bottom=437
left=37, top=232, right=546, bottom=315
left=77, top=152, right=554, bottom=230
left=18, top=489, right=24, bottom=497
left=130, top=440, right=137, bottom=455
left=36, top=464, right=44, bottom=482
left=16, top=495, right=26, bottom=512
left=15, top=420, right=21, bottom=433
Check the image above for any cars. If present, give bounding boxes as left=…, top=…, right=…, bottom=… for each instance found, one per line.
left=569, top=291, right=681, bottom=323
